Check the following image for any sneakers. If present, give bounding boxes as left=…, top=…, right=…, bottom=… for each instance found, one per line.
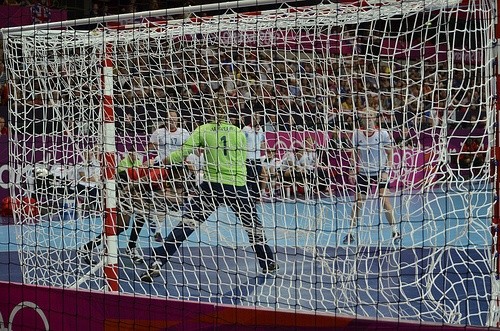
left=262, top=263, right=279, bottom=273
left=392, top=233, right=402, bottom=242
left=343, top=234, right=354, bottom=244
left=125, top=246, right=144, bottom=264
left=140, top=264, right=162, bottom=282
left=76, top=246, right=98, bottom=267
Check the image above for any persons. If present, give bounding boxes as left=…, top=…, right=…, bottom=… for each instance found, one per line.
left=0, top=0, right=492, bottom=238
left=343, top=106, right=403, bottom=245
left=76, top=162, right=196, bottom=265
left=140, top=96, right=280, bottom=283
